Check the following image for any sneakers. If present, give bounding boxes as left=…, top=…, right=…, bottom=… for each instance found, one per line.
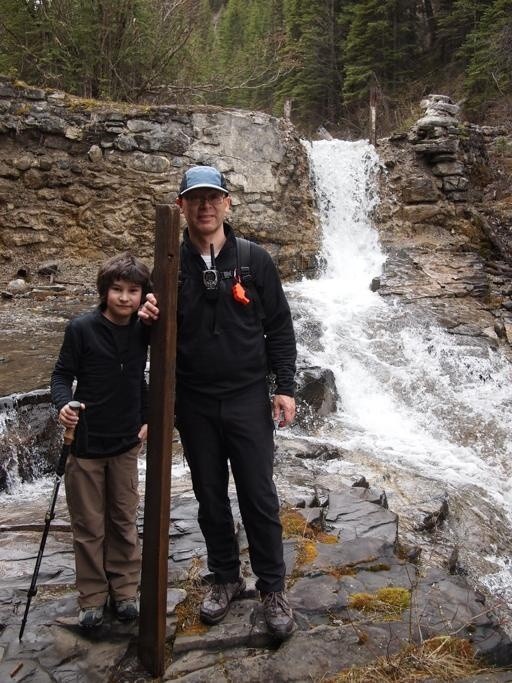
left=109, top=596, right=140, bottom=623
left=198, top=567, right=248, bottom=625
left=259, top=587, right=296, bottom=639
left=78, top=599, right=108, bottom=630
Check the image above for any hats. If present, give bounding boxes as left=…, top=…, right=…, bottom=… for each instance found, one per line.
left=178, top=166, right=230, bottom=197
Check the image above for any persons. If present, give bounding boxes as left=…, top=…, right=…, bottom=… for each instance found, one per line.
left=50, top=256, right=150, bottom=632
left=136, top=164, right=299, bottom=644
